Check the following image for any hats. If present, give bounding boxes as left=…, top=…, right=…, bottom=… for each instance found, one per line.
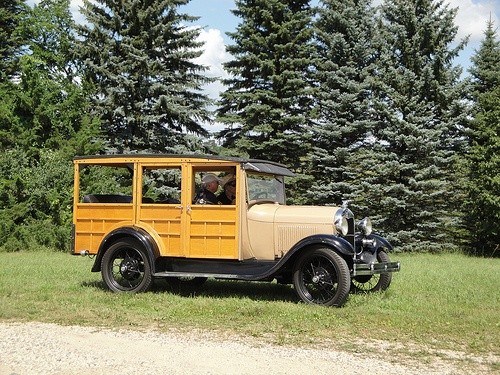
left=221, top=173, right=236, bottom=189
left=202, top=174, right=224, bottom=184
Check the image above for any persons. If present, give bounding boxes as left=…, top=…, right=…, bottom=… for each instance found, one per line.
left=216, top=173, right=237, bottom=204
left=192, top=173, right=223, bottom=205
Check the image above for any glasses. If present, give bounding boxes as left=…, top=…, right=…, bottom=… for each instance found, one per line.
left=217, top=181, right=221, bottom=185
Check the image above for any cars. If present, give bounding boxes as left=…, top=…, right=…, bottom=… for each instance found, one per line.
left=69, top=153, right=402, bottom=310
